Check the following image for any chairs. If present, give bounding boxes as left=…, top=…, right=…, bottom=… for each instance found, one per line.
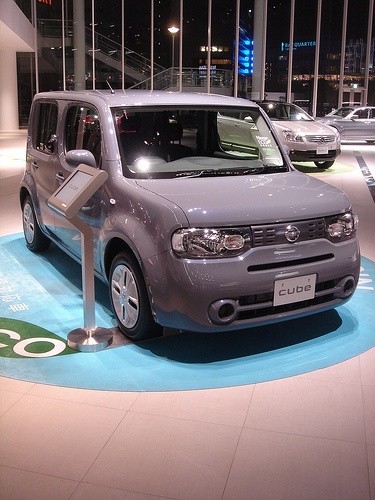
left=120, top=116, right=150, bottom=164
left=153, top=123, right=193, bottom=162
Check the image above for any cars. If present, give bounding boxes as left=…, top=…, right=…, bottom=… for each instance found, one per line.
left=19, top=89, right=361, bottom=342
left=318, top=106, right=375, bottom=144
left=217, top=99, right=342, bottom=169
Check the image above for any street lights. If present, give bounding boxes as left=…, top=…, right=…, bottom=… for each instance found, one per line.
left=167, top=25, right=180, bottom=86
